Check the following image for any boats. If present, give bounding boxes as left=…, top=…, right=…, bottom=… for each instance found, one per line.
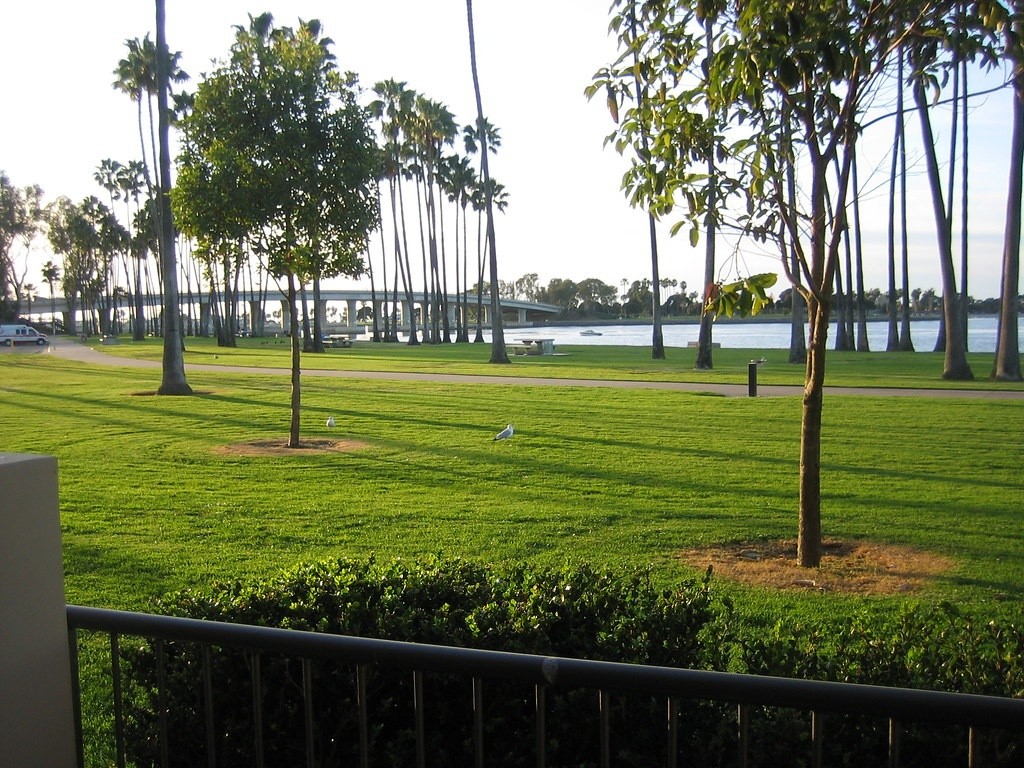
left=579, top=329, right=602, bottom=336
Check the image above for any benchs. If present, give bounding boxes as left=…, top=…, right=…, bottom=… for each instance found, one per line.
left=322, top=333, right=352, bottom=348
left=505, top=338, right=554, bottom=355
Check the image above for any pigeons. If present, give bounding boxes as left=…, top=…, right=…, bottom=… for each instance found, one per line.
left=491, top=424, right=514, bottom=441
left=326, top=416, right=335, bottom=428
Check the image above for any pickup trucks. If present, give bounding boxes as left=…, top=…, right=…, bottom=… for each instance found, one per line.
left=0, top=324, right=49, bottom=347
